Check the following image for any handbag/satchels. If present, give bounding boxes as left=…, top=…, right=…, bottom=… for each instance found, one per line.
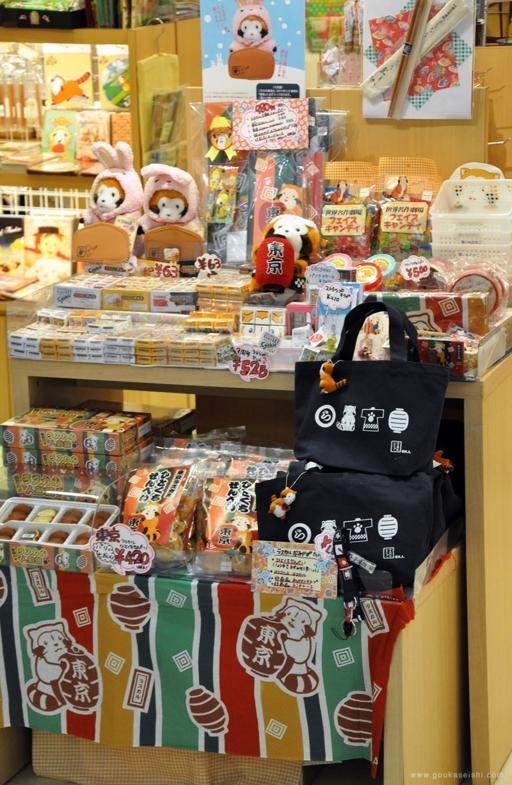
left=292, top=301, right=449, bottom=479
left=255, top=454, right=457, bottom=590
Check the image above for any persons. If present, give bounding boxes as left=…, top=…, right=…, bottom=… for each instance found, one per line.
left=25, top=225, right=72, bottom=285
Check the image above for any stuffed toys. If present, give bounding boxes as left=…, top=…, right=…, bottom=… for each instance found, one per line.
left=78, top=140, right=144, bottom=262
left=432, top=450, right=454, bottom=475
left=318, top=359, right=348, bottom=394
left=268, top=487, right=296, bottom=521
left=222, top=2, right=282, bottom=82
left=238, top=213, right=328, bottom=293
left=130, top=162, right=205, bottom=263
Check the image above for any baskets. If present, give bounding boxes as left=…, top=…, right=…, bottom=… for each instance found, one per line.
left=426, top=161, right=512, bottom=277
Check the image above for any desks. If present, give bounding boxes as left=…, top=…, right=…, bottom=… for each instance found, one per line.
left=7, top=273, right=511, bottom=785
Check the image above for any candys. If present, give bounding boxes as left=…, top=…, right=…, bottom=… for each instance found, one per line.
left=316, top=194, right=432, bottom=263
